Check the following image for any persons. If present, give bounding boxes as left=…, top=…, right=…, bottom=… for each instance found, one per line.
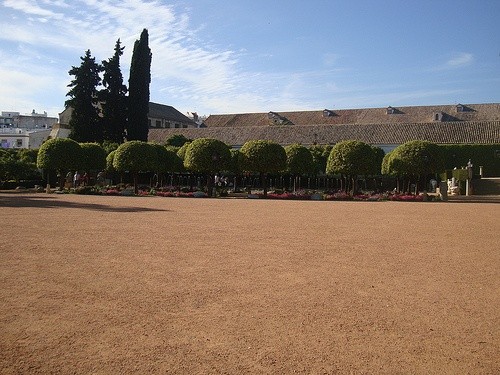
left=215, top=174, right=237, bottom=194
left=57, top=170, right=88, bottom=188
left=97, top=172, right=104, bottom=187
